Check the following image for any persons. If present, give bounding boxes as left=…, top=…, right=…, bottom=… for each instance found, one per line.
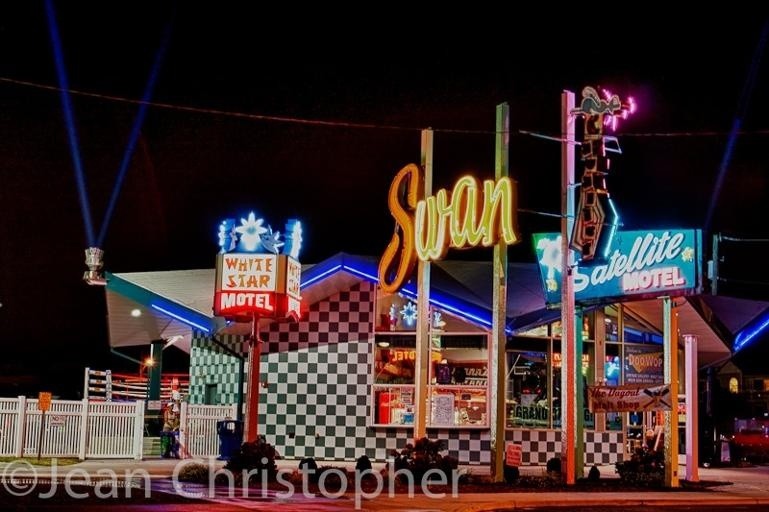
left=162, top=402, right=175, bottom=431
